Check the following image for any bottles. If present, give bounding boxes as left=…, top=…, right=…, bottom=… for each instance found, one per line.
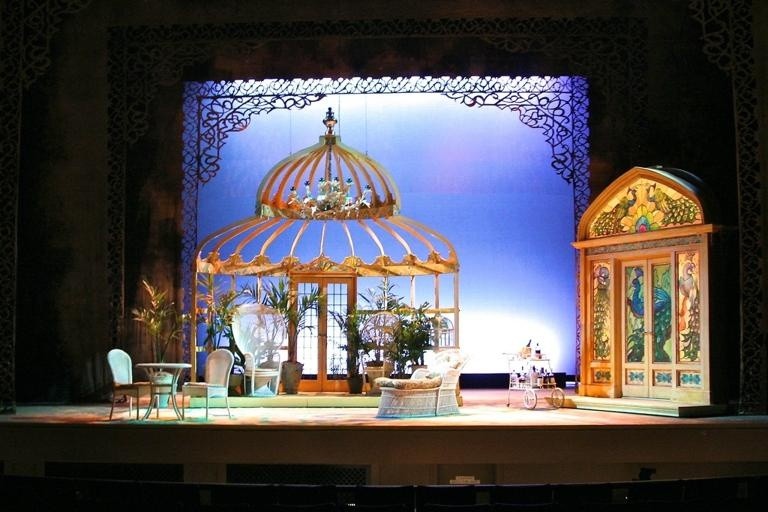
left=510, top=365, right=555, bottom=389
left=527, top=339, right=532, bottom=347
left=535, top=343, right=542, bottom=359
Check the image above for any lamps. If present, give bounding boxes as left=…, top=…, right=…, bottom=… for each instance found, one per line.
left=286, top=93, right=373, bottom=218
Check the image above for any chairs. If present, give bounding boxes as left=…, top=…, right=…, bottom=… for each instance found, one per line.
left=106, top=348, right=235, bottom=422
left=373, top=348, right=467, bottom=420
